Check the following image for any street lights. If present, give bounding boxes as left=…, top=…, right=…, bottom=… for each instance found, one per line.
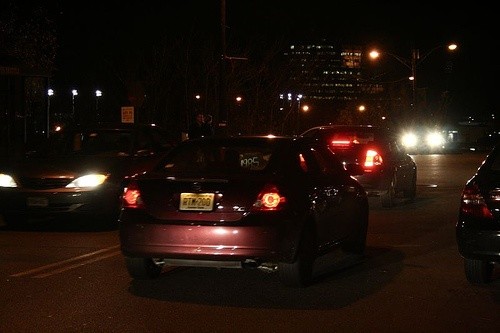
left=95, top=90, right=103, bottom=111
left=71, top=88, right=78, bottom=113
left=47, top=88, right=55, bottom=135
left=369, top=43, right=458, bottom=107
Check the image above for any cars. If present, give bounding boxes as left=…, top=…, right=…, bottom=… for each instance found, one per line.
left=0, top=126, right=167, bottom=235
left=455, top=145, right=500, bottom=283
left=116, top=133, right=370, bottom=288
left=295, top=124, right=418, bottom=208
